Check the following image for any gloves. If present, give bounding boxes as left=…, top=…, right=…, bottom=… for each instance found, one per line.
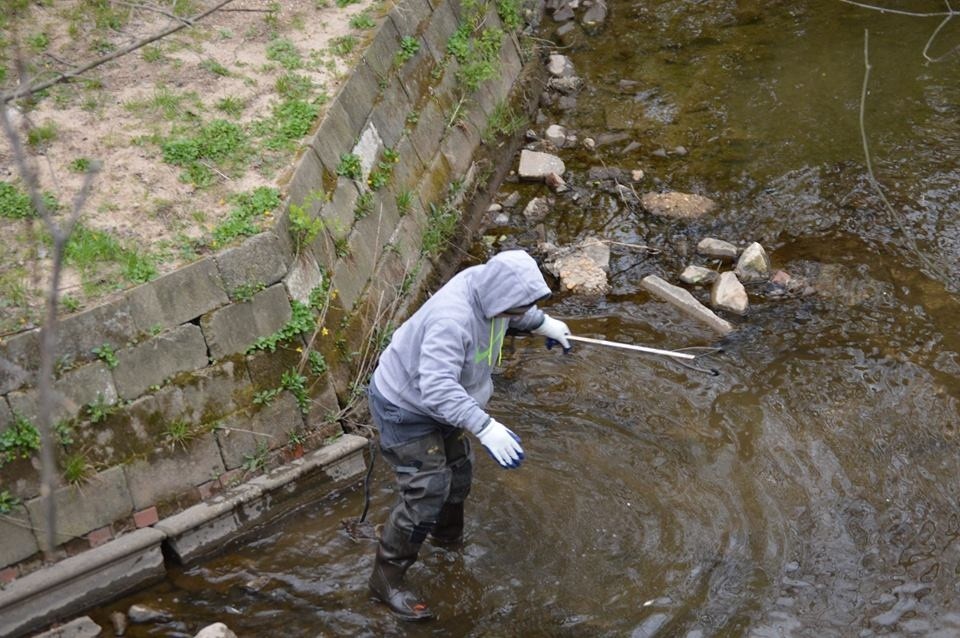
left=475, top=417, right=525, bottom=470
left=530, top=313, right=572, bottom=355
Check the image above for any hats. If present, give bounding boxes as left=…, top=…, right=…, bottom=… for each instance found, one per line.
left=504, top=300, right=537, bottom=315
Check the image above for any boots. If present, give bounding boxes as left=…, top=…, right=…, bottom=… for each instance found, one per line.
left=368, top=431, right=452, bottom=622
left=430, top=425, right=472, bottom=549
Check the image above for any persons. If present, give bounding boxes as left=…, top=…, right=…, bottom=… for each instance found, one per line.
left=368, top=249, right=571, bottom=623
